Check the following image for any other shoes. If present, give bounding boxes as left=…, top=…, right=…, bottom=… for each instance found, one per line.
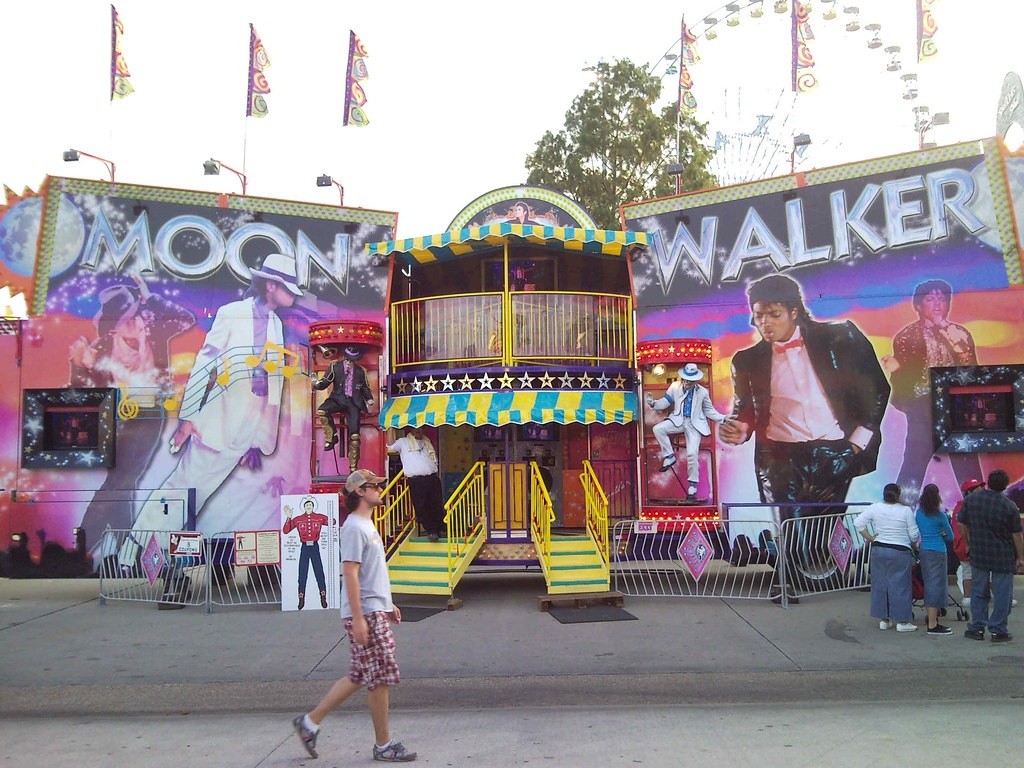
left=1011, top=599, right=1018, bottom=607
left=962, top=597, right=970, bottom=608
left=118, top=536, right=138, bottom=572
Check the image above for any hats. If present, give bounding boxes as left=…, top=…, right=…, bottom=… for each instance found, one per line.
left=249, top=253, right=305, bottom=298
left=345, top=469, right=388, bottom=494
left=961, top=478, right=986, bottom=493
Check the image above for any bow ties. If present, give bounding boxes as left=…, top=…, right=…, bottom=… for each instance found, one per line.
left=774, top=337, right=803, bottom=355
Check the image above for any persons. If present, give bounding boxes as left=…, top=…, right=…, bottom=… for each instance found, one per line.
left=951, top=469, right=1024, bottom=642
left=309, top=345, right=375, bottom=477
left=469, top=202, right=568, bottom=227
left=718, top=273, right=893, bottom=565
left=879, top=278, right=984, bottom=507
left=68, top=274, right=195, bottom=556
left=853, top=483, right=919, bottom=632
left=119, top=253, right=304, bottom=577
left=386, top=424, right=448, bottom=542
left=291, top=468, right=417, bottom=763
left=728, top=527, right=779, bottom=568
left=915, top=483, right=955, bottom=635
left=646, top=362, right=739, bottom=501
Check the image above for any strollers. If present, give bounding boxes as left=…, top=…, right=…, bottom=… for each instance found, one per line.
left=912, top=547, right=971, bottom=626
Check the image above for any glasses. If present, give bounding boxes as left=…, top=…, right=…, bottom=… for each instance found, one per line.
left=360, top=482, right=384, bottom=492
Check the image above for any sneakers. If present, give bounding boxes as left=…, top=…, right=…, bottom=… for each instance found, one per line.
left=292, top=713, right=321, bottom=759
left=964, top=628, right=985, bottom=640
left=927, top=624, right=953, bottom=635
left=429, top=533, right=439, bottom=542
left=896, top=622, right=918, bottom=632
left=438, top=530, right=447, bottom=538
left=372, top=740, right=417, bottom=762
left=991, top=632, right=1014, bottom=642
left=880, top=621, right=893, bottom=630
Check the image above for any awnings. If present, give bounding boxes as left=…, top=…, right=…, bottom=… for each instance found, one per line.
left=379, top=390, right=640, bottom=433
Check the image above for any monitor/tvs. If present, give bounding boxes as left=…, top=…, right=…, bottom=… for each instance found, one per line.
left=474, top=425, right=513, bottom=442
left=517, top=422, right=559, bottom=442
